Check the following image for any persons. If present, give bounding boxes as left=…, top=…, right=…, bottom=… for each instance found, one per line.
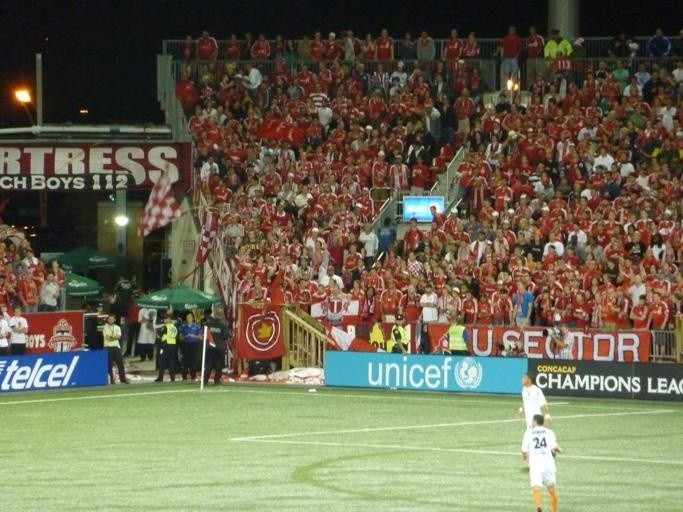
left=0, top=227, right=66, bottom=356
left=516, top=372, right=551, bottom=472
left=521, top=414, right=563, bottom=511
left=102, top=272, right=178, bottom=385
left=175, top=30, right=682, bottom=385
